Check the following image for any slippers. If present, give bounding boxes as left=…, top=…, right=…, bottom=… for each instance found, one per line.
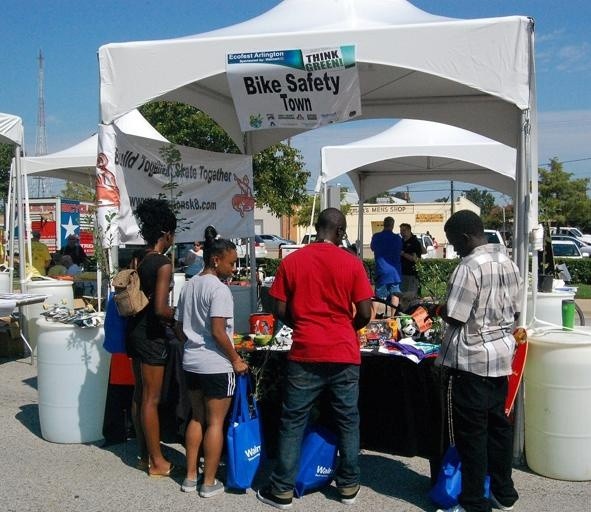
left=137, top=453, right=187, bottom=476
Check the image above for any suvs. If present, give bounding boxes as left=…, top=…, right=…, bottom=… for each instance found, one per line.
left=550, top=225, right=591, bottom=243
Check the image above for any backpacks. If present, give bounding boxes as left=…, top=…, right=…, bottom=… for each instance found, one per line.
left=110, top=251, right=155, bottom=316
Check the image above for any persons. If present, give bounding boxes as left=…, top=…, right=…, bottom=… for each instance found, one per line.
left=124, top=200, right=182, bottom=476
left=436, top=211, right=526, bottom=511
left=398, top=223, right=421, bottom=296
left=24, top=231, right=87, bottom=276
left=370, top=215, right=404, bottom=307
left=170, top=224, right=248, bottom=500
left=257, top=208, right=373, bottom=510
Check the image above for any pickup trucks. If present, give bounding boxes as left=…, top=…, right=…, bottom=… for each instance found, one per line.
left=279, top=232, right=352, bottom=260
left=442, top=229, right=509, bottom=261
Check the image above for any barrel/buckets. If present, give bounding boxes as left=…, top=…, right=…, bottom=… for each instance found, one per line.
left=35, top=313, right=113, bottom=444
left=1, top=271, right=10, bottom=294
left=524, top=326, right=591, bottom=482
left=527, top=292, right=575, bottom=326
left=26, top=281, right=74, bottom=353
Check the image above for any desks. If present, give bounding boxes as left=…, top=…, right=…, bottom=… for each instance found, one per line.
left=238, top=345, right=447, bottom=486
left=0, top=294, right=48, bottom=365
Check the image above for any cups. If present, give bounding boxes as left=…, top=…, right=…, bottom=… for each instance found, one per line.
left=562, top=299, right=575, bottom=331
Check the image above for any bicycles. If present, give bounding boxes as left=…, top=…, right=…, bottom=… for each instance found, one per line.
left=549, top=268, right=585, bottom=328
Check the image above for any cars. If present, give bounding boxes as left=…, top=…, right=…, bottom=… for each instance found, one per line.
left=529, top=240, right=590, bottom=262
left=229, top=233, right=268, bottom=262
left=527, top=235, right=591, bottom=258
left=397, top=231, right=438, bottom=261
left=255, top=234, right=296, bottom=252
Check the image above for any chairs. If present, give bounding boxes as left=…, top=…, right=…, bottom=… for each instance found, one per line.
left=82, top=278, right=108, bottom=312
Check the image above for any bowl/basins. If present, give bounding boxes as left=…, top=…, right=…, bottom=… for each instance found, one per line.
left=233, top=333, right=273, bottom=346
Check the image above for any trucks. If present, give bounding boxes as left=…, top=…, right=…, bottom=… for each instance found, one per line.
left=6, top=196, right=197, bottom=270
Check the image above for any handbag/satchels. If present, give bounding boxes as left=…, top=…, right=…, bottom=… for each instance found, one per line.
left=228, top=414, right=262, bottom=490
left=430, top=443, right=490, bottom=507
left=293, top=425, right=336, bottom=498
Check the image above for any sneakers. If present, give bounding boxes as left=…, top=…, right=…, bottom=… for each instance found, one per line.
left=180, top=474, right=201, bottom=491
left=341, top=483, right=360, bottom=504
left=201, top=479, right=224, bottom=498
left=257, top=483, right=293, bottom=508
